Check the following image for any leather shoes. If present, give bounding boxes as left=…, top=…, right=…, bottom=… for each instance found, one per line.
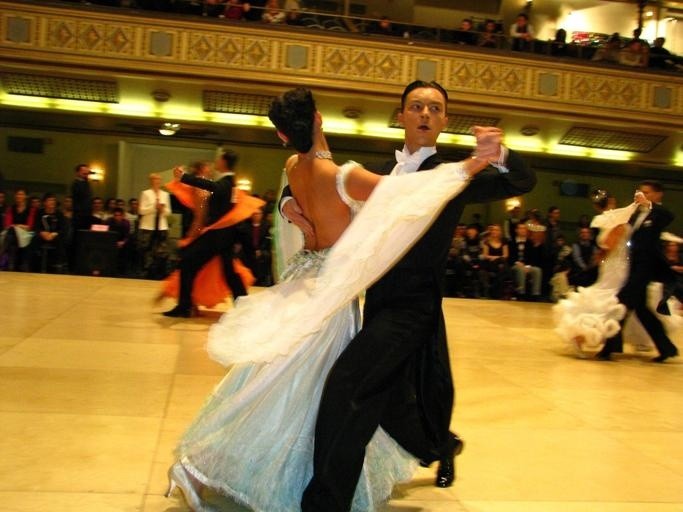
left=655, top=300, right=671, bottom=315
left=598, top=344, right=624, bottom=358
left=433, top=436, right=463, bottom=487
left=162, top=304, right=193, bottom=320
left=651, top=347, right=677, bottom=362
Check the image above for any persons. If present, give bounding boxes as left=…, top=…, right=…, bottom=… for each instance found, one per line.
left=569, top=185, right=643, bottom=361
left=590, top=178, right=679, bottom=363
left=274, top=80, right=538, bottom=512
left=151, top=158, right=213, bottom=317
left=161, top=145, right=247, bottom=317
left=196, top=1, right=683, bottom=76
left=163, top=84, right=503, bottom=512
left=1, top=165, right=683, bottom=305
